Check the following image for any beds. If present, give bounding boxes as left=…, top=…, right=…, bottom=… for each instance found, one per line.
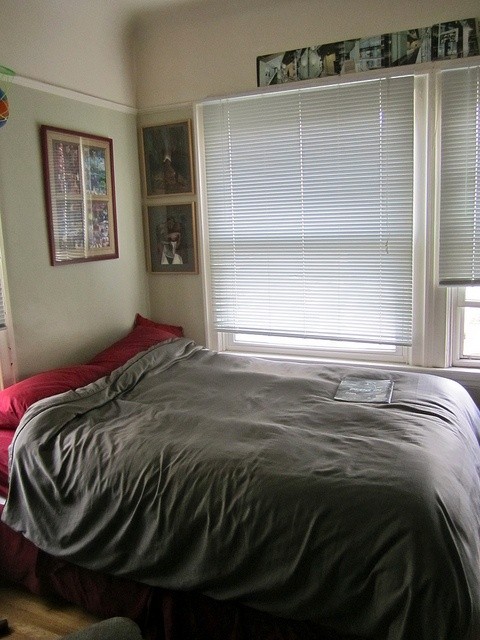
left=2, top=339, right=478, bottom=640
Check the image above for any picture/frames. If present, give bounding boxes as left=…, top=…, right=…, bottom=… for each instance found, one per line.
left=140, top=198, right=199, bottom=276
left=39, top=123, right=119, bottom=267
left=136, top=118, right=194, bottom=198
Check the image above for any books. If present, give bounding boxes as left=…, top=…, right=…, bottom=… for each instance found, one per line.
left=334, top=376, right=394, bottom=404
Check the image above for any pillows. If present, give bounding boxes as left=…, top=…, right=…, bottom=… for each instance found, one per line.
left=2, top=365, right=109, bottom=428
left=89, top=312, right=183, bottom=374
